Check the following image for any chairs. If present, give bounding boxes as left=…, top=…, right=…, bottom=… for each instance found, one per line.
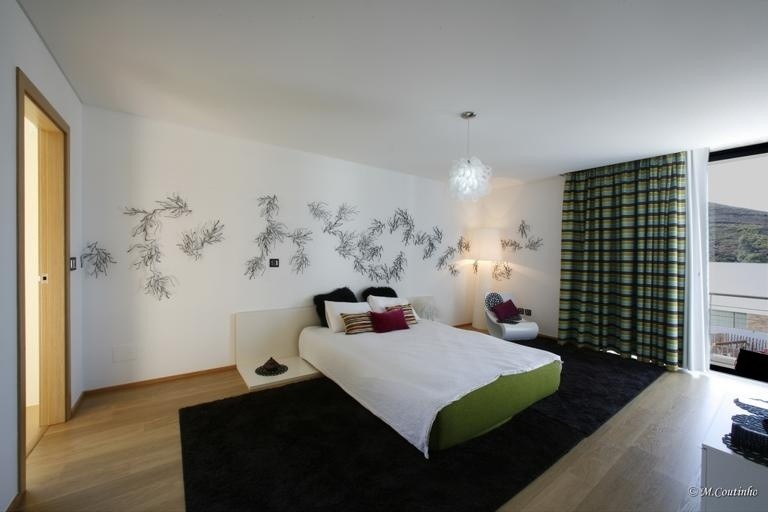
left=484, top=290, right=539, bottom=341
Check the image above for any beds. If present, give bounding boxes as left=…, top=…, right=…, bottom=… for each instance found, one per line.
left=300, top=318, right=562, bottom=460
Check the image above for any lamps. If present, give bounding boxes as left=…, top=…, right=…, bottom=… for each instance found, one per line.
left=449, top=111, right=491, bottom=200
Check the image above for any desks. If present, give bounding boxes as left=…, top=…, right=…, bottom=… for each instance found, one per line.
left=701, top=395, right=768, bottom=512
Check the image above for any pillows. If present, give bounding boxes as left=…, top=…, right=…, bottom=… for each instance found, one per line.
left=492, top=299, right=519, bottom=320
left=313, top=287, right=418, bottom=334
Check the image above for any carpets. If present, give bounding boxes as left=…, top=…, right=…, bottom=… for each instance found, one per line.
left=179, top=333, right=666, bottom=512
